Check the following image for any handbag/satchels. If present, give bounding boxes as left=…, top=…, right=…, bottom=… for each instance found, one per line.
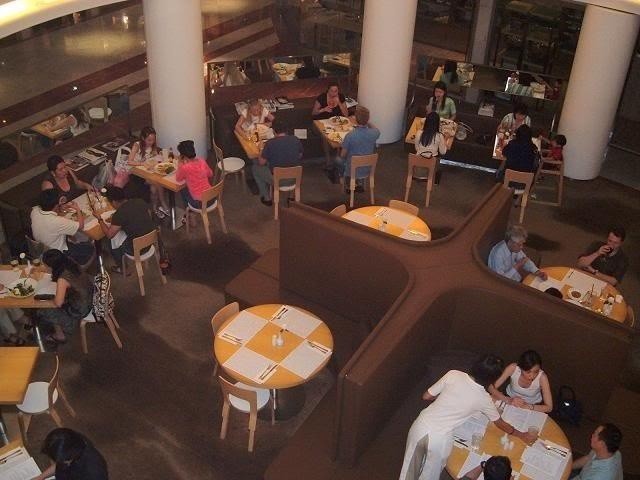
left=455, top=123, right=473, bottom=143
left=549, top=386, right=580, bottom=423
left=483, top=355, right=503, bottom=371
left=479, top=135, right=496, bottom=145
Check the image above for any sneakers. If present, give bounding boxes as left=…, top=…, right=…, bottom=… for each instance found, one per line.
left=261, top=196, right=272, bottom=205
left=112, top=265, right=127, bottom=274
left=160, top=260, right=169, bottom=268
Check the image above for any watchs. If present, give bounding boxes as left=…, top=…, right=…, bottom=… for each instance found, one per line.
left=98, top=219, right=103, bottom=224
left=40, top=474, right=45, bottom=480
left=592, top=270, right=599, bottom=275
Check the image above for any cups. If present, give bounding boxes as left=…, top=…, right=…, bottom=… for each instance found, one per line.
left=10, top=260, right=20, bottom=272
left=244, top=127, right=251, bottom=140
left=584, top=290, right=597, bottom=307
left=472, top=432, right=482, bottom=454
left=159, top=149, right=168, bottom=162
left=527, top=425, right=539, bottom=446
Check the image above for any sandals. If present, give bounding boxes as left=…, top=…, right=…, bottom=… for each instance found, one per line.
left=4, top=335, right=24, bottom=346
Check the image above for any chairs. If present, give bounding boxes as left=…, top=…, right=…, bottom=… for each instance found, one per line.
left=343, top=154, right=377, bottom=208
left=266, top=166, right=305, bottom=220
left=501, top=170, right=533, bottom=225
left=80, top=256, right=123, bottom=353
left=16, top=353, right=76, bottom=433
left=24, top=234, right=43, bottom=258
left=532, top=158, right=566, bottom=206
left=217, top=376, right=274, bottom=452
left=206, top=299, right=239, bottom=379
left=327, top=205, right=347, bottom=217
left=209, top=140, right=246, bottom=194
left=404, top=153, right=435, bottom=209
left=389, top=199, right=419, bottom=215
left=628, top=305, right=635, bottom=330
left=122, top=229, right=167, bottom=296
left=13, top=131, right=37, bottom=156
left=184, top=179, right=229, bottom=245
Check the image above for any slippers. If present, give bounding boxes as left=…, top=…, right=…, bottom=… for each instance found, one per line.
left=153, top=210, right=164, bottom=218
left=45, top=333, right=67, bottom=345
left=160, top=207, right=169, bottom=214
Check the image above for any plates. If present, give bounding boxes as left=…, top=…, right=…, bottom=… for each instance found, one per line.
left=153, top=162, right=178, bottom=175
left=328, top=115, right=351, bottom=128
left=7, top=278, right=38, bottom=299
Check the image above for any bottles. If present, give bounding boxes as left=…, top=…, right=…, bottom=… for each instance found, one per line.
left=168, top=147, right=174, bottom=163
left=253, top=125, right=259, bottom=142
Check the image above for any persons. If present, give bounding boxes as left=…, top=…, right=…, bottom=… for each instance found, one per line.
left=333, top=106, right=380, bottom=194
left=488, top=351, right=553, bottom=412
left=42, top=155, right=94, bottom=201
left=173, top=140, right=217, bottom=228
left=459, top=455, right=515, bottom=480
left=546, top=79, right=563, bottom=100
left=488, top=225, right=548, bottom=282
left=439, top=61, right=463, bottom=93
left=0, top=283, right=34, bottom=345
left=127, top=126, right=170, bottom=218
left=41, top=107, right=91, bottom=136
left=30, top=427, right=109, bottom=480
left=31, top=189, right=98, bottom=276
left=415, top=111, right=447, bottom=186
left=576, top=227, right=629, bottom=286
left=426, top=81, right=457, bottom=120
left=536, top=134, right=566, bottom=184
left=252, top=117, right=303, bottom=208
left=312, top=82, right=348, bottom=170
left=507, top=72, right=534, bottom=97
left=544, top=287, right=563, bottom=299
left=571, top=423, right=623, bottom=480
left=293, top=56, right=321, bottom=80
left=399, top=357, right=539, bottom=480
left=40, top=249, right=94, bottom=344
left=502, top=124, right=541, bottom=199
left=492, top=103, right=531, bottom=179
left=235, top=100, right=275, bottom=140
left=92, top=186, right=173, bottom=275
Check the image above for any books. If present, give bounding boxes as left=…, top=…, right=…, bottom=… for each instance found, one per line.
left=102, top=135, right=130, bottom=152
left=0, top=446, right=46, bottom=480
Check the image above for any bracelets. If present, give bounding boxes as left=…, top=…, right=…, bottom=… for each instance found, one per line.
left=509, top=426, right=514, bottom=435
left=529, top=404, right=534, bottom=410
left=140, top=161, right=142, bottom=166
